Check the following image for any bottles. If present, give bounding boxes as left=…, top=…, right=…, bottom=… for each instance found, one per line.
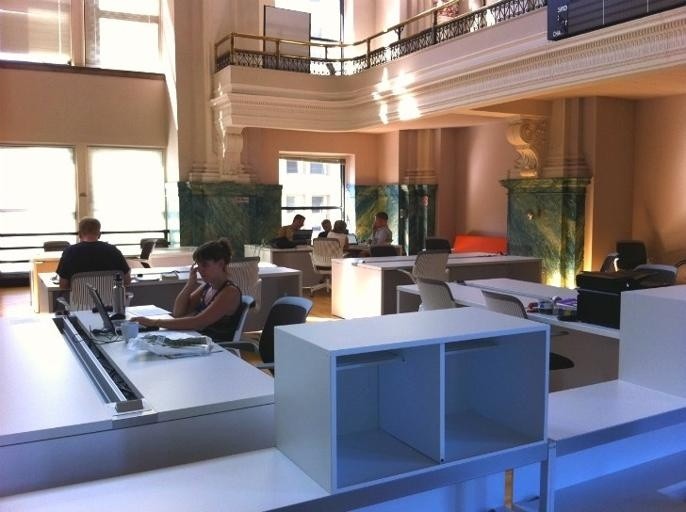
left=112, top=273, right=125, bottom=314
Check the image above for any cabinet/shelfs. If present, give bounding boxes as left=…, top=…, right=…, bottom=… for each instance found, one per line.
left=275, top=307, right=550, bottom=495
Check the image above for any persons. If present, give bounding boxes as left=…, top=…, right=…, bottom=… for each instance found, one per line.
left=327, top=220, right=349, bottom=254
left=367, top=212, right=392, bottom=246
left=56, top=218, right=131, bottom=288
left=129, top=237, right=242, bottom=342
left=279, top=214, right=305, bottom=241
left=318, top=220, right=331, bottom=237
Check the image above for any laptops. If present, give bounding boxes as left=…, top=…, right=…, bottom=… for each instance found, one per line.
left=85, top=281, right=159, bottom=337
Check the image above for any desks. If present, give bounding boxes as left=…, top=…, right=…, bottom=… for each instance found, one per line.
left=37, top=261, right=303, bottom=313
left=0, top=304, right=281, bottom=497
left=395, top=274, right=622, bottom=393
left=245, top=242, right=402, bottom=285
left=331, top=251, right=544, bottom=321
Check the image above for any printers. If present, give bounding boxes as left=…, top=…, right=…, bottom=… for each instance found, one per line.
left=575, top=240, right=676, bottom=329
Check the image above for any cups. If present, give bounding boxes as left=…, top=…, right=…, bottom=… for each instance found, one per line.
left=115, top=322, right=139, bottom=344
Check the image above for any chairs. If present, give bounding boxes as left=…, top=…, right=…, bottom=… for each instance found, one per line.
left=414, top=277, right=454, bottom=312
left=215, top=295, right=312, bottom=366
left=56, top=271, right=123, bottom=311
left=310, top=237, right=352, bottom=297
left=480, top=290, right=530, bottom=320
left=396, top=250, right=449, bottom=287
left=216, top=296, right=255, bottom=359
